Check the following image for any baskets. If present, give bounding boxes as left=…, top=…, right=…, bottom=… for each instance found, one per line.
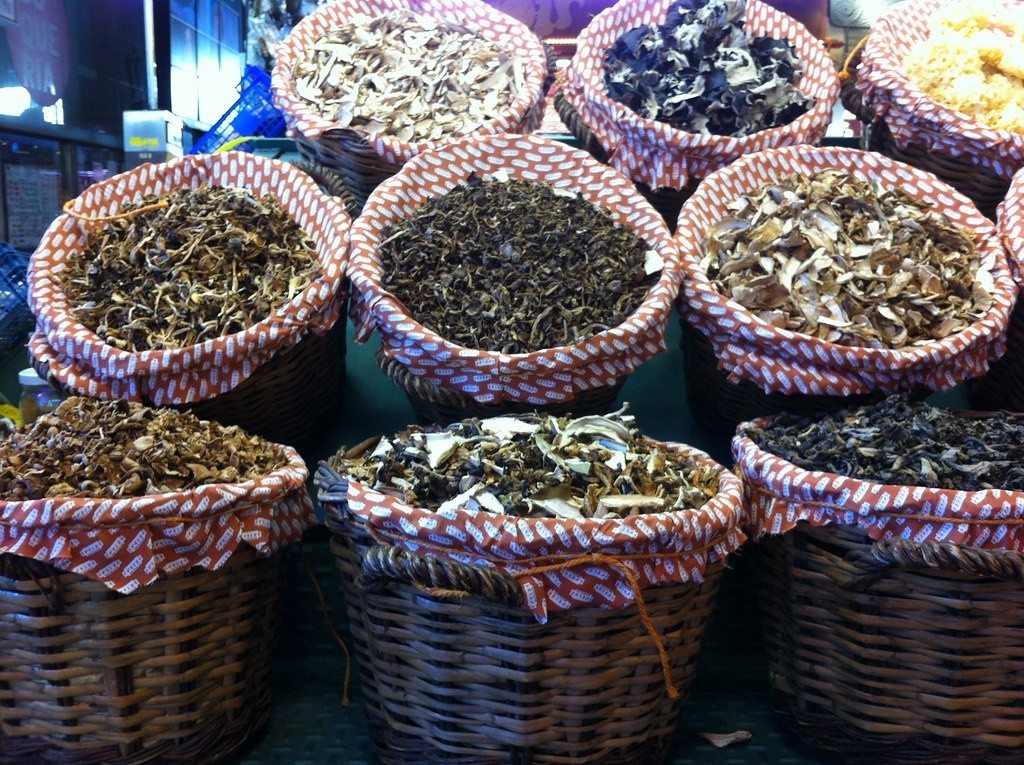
left=0, top=242, right=36, bottom=359
left=839, top=0, right=1024, bottom=220
left=1, top=443, right=311, bottom=765
left=732, top=405, right=1024, bottom=764
left=190, top=66, right=284, bottom=153
left=351, top=133, right=677, bottom=423
left=673, top=144, right=1017, bottom=435
left=314, top=414, right=745, bottom=765
left=554, top=0, right=840, bottom=235
left=272, top=0, right=557, bottom=208
left=29, top=151, right=350, bottom=446
